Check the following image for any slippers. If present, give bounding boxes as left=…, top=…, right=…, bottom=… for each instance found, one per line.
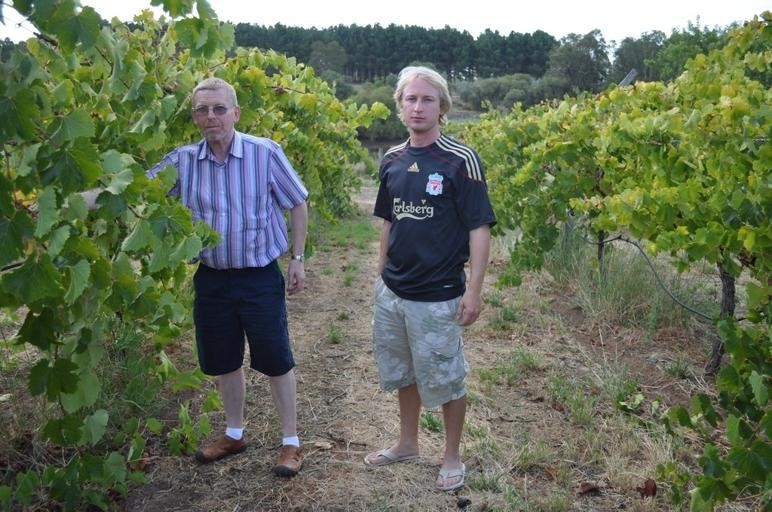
left=436, top=463, right=465, bottom=490
left=364, top=448, right=420, bottom=466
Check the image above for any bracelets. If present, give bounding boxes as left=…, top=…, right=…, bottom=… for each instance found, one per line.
left=291, top=254, right=306, bottom=264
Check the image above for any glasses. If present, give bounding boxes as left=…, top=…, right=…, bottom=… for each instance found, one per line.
left=192, top=106, right=236, bottom=114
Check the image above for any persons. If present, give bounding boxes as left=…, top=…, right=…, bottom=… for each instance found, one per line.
left=32, top=77, right=309, bottom=481
left=364, top=65, right=497, bottom=494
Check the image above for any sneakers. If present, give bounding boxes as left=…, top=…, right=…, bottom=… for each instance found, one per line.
left=196, top=431, right=246, bottom=463
left=273, top=445, right=304, bottom=476
